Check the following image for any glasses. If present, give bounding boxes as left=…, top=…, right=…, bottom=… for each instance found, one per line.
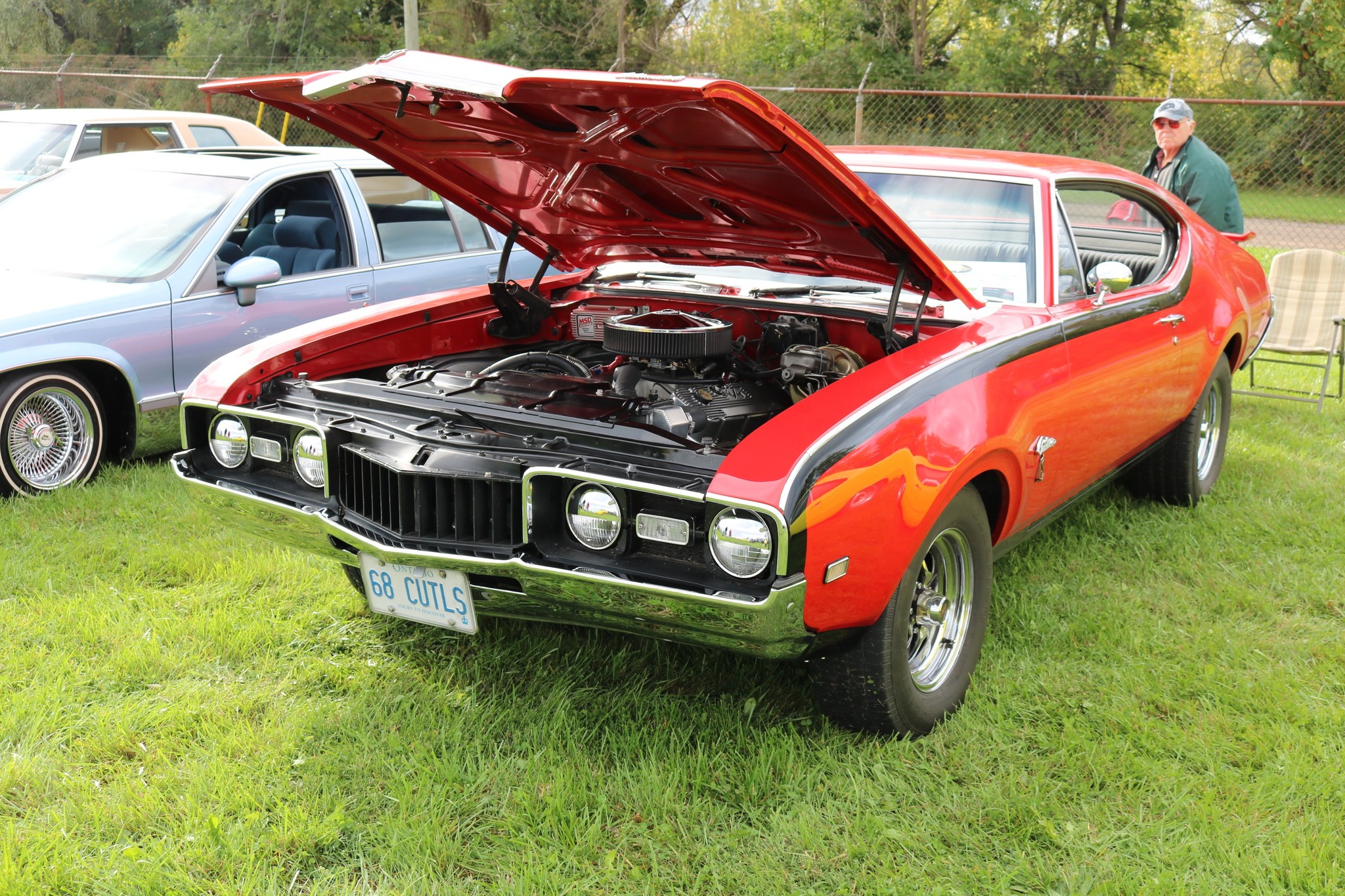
left=1152, top=119, right=1190, bottom=129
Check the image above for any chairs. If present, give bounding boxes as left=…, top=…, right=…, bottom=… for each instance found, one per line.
left=1229, top=248, right=1345, bottom=413
left=242, top=210, right=338, bottom=274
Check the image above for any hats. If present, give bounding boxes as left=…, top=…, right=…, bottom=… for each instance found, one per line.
left=1150, top=98, right=1194, bottom=125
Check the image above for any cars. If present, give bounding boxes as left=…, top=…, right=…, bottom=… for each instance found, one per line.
left=168, top=49, right=1276, bottom=740
left=1, top=108, right=285, bottom=193
left=1, top=144, right=582, bottom=501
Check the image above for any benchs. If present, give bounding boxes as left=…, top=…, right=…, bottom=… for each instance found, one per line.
left=285, top=200, right=450, bottom=223
left=922, top=238, right=1156, bottom=303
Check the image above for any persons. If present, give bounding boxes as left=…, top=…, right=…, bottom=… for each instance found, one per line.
left=1105, top=97, right=1245, bottom=247
